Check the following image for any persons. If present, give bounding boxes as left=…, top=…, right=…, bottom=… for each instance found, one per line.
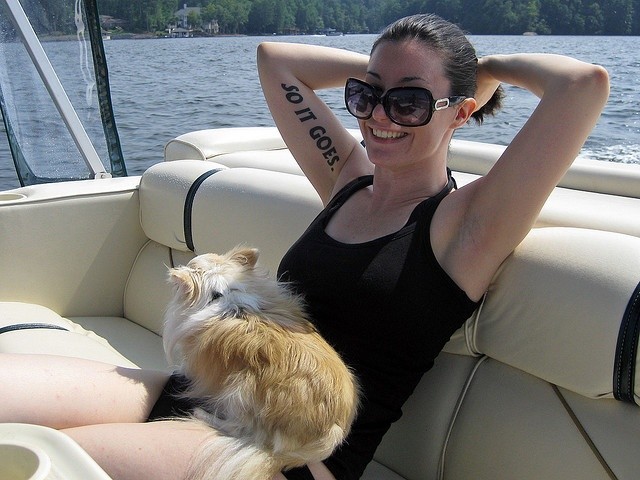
left=0, top=12, right=611, bottom=477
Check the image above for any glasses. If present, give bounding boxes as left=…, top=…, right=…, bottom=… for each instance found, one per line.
left=344, top=76, right=467, bottom=127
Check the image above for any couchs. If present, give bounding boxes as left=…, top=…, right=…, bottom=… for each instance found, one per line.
left=0, top=124, right=640, bottom=479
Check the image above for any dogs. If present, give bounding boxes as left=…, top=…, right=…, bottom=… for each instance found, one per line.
left=164, top=245, right=365, bottom=480
